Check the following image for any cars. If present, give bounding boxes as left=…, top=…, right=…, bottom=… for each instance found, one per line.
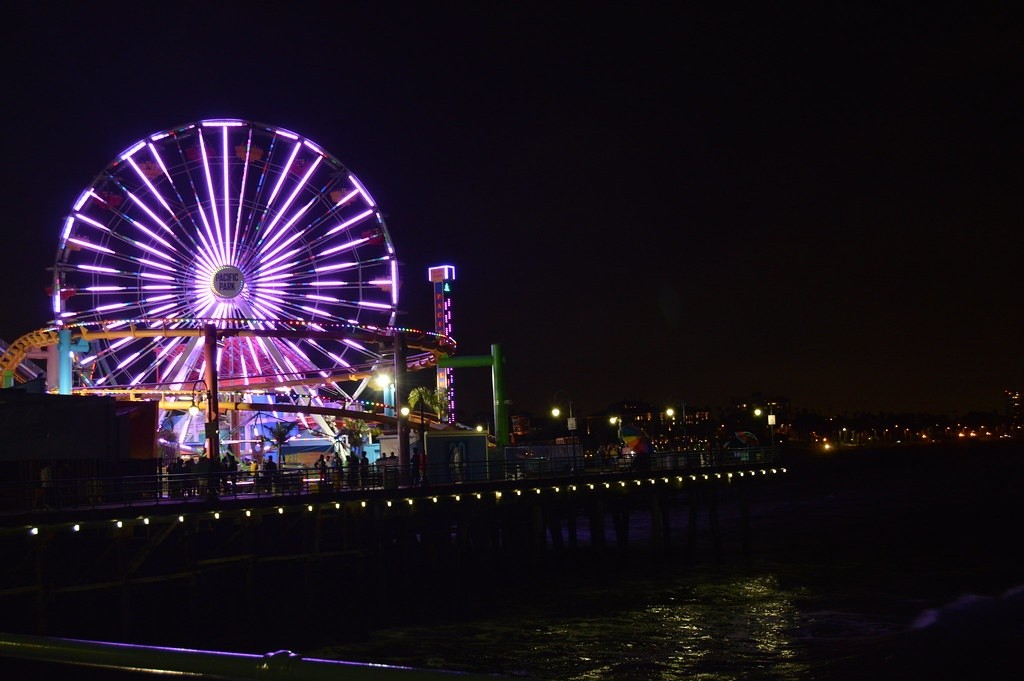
left=808, top=432, right=830, bottom=448
left=999, top=432, right=1011, bottom=441
left=867, top=436, right=880, bottom=441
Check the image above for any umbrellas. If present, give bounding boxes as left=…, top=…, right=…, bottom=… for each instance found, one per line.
left=734, top=430, right=761, bottom=448
left=621, top=426, right=654, bottom=455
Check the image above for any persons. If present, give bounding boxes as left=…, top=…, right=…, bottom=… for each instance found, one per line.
left=379, top=452, right=397, bottom=460
left=410, top=447, right=420, bottom=489
left=454, top=446, right=464, bottom=485
left=169, top=453, right=239, bottom=498
left=333, top=451, right=361, bottom=492
left=324, top=456, right=333, bottom=483
left=359, top=451, right=368, bottom=489
left=314, top=454, right=327, bottom=483
left=263, top=455, right=277, bottom=494
left=594, top=445, right=619, bottom=473
left=251, top=460, right=260, bottom=478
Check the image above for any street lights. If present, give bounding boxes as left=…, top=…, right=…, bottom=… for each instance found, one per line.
left=550, top=389, right=574, bottom=444
left=754, top=408, right=763, bottom=447
left=885, top=429, right=889, bottom=440
left=665, top=407, right=676, bottom=451
left=191, top=379, right=213, bottom=460
left=608, top=416, right=622, bottom=446
left=894, top=424, right=992, bottom=441
left=767, top=401, right=777, bottom=457
left=842, top=428, right=847, bottom=441
left=409, top=387, right=427, bottom=479
